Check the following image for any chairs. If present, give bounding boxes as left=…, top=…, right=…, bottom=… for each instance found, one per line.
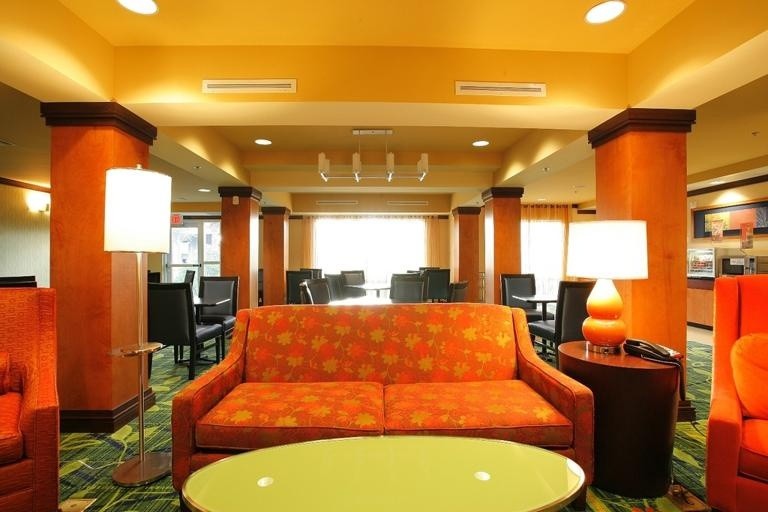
left=148, top=268, right=565, bottom=381
left=701, top=267, right=767, bottom=512
left=1, top=276, right=37, bottom=288
left=0, top=281, right=66, bottom=511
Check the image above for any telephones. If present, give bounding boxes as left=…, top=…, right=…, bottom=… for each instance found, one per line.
left=623, top=338, right=684, bottom=362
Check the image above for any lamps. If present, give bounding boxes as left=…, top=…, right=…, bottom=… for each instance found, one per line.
left=563, top=216, right=650, bottom=352
left=315, top=128, right=430, bottom=184
left=103, top=159, right=175, bottom=490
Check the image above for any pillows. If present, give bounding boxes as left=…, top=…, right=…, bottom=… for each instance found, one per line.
left=727, top=330, right=767, bottom=421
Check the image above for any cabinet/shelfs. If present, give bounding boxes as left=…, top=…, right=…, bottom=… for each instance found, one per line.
left=557, top=341, right=677, bottom=498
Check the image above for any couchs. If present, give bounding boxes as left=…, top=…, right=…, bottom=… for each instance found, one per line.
left=168, top=300, right=597, bottom=504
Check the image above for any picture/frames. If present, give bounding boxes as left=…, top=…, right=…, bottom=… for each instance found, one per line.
left=687, top=195, right=767, bottom=242
